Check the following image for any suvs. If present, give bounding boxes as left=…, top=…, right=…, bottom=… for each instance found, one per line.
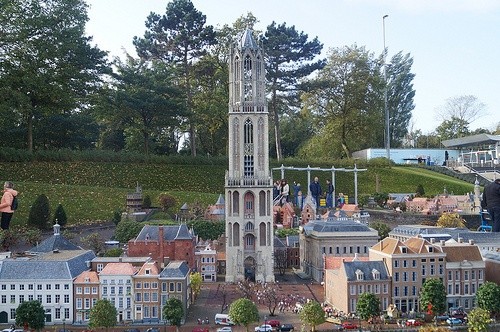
left=255, top=324, right=273, bottom=332
left=276, top=324, right=295, bottom=332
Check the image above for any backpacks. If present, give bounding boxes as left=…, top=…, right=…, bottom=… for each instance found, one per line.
left=8, top=192, right=18, bottom=210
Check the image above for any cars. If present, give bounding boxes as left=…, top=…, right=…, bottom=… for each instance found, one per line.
left=262, top=320, right=280, bottom=328
left=342, top=322, right=358, bottom=330
left=395, top=314, right=469, bottom=328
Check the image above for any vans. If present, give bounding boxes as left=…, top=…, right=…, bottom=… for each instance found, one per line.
left=218, top=327, right=232, bottom=332
left=214, top=313, right=237, bottom=327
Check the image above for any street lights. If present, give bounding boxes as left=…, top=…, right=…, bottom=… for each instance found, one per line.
left=383, top=14, right=390, bottom=159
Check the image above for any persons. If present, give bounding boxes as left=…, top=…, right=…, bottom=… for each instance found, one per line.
left=292, top=180, right=301, bottom=206
left=480, top=179, right=500, bottom=231
left=0, top=181, right=19, bottom=230
left=309, top=177, right=322, bottom=207
left=274, top=180, right=289, bottom=206
left=325, top=180, right=335, bottom=208
